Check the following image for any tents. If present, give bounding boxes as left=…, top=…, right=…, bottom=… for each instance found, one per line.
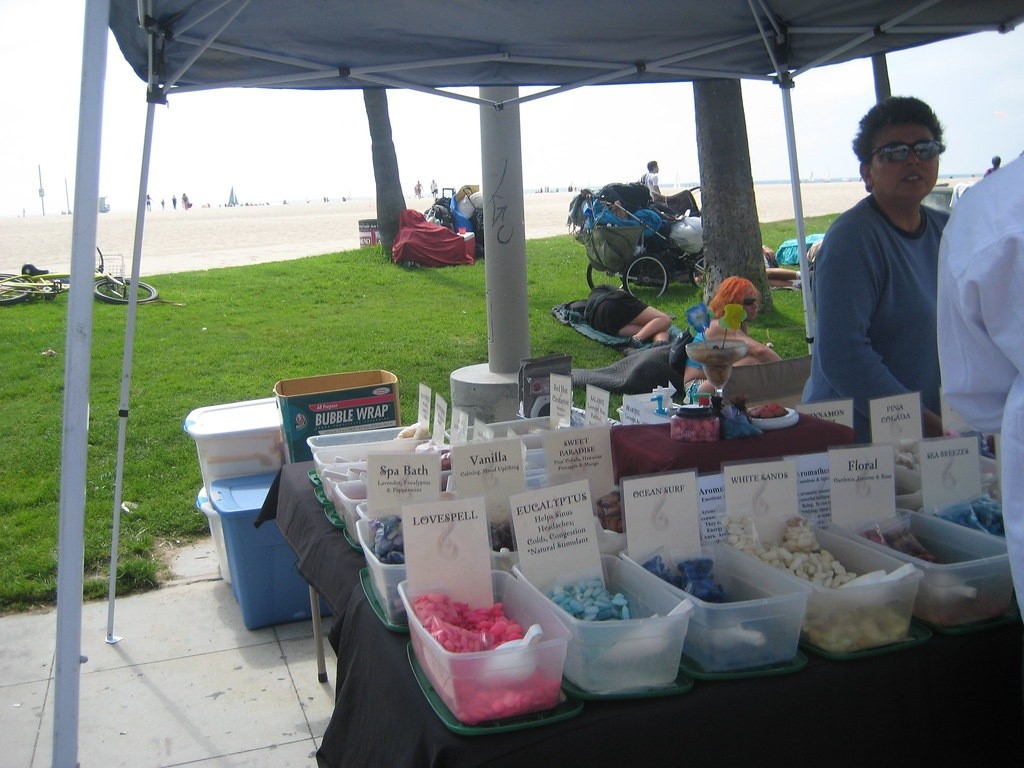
left=52, top=1, right=1024, bottom=768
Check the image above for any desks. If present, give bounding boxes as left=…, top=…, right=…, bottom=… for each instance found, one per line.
left=254, top=464, right=1023, bottom=768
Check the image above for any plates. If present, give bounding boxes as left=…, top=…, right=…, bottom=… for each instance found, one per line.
left=749, top=405, right=800, bottom=431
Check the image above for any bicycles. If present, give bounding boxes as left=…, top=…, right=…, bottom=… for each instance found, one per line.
left=0, top=247, right=159, bottom=305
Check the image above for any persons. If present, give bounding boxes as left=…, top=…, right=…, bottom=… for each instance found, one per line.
left=160, top=198, right=165, bottom=209
left=801, top=96, right=950, bottom=439
left=323, top=196, right=329, bottom=203
left=414, top=180, right=439, bottom=199
left=206, top=200, right=289, bottom=208
left=146, top=194, right=154, bottom=211
left=640, top=161, right=661, bottom=201
left=937, top=150, right=1024, bottom=624
left=171, top=194, right=177, bottom=209
left=341, top=196, right=346, bottom=201
left=983, top=156, right=1001, bottom=178
left=762, top=245, right=814, bottom=290
left=584, top=284, right=673, bottom=349
left=181, top=192, right=190, bottom=211
left=683, top=275, right=783, bottom=395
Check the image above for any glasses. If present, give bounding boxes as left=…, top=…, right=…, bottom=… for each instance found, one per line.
left=735, top=298, right=756, bottom=305
left=866, top=139, right=946, bottom=163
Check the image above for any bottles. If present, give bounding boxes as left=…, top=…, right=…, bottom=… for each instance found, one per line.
left=669, top=405, right=720, bottom=442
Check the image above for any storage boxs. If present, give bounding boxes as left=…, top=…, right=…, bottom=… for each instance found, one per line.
left=197, top=486, right=231, bottom=585
left=305, top=411, right=1012, bottom=727
left=274, top=370, right=401, bottom=464
left=457, top=230, right=476, bottom=261
left=182, top=397, right=288, bottom=501
left=210, top=469, right=333, bottom=632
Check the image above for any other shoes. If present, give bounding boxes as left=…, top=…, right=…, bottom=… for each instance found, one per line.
left=629, top=335, right=643, bottom=349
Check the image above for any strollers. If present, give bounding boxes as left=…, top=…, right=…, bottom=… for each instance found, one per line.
left=567, top=186, right=705, bottom=301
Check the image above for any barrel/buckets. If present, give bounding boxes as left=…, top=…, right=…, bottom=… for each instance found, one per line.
left=358, top=218, right=382, bottom=248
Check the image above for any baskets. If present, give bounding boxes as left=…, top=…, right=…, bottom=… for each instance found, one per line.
left=98, top=254, right=126, bottom=277
